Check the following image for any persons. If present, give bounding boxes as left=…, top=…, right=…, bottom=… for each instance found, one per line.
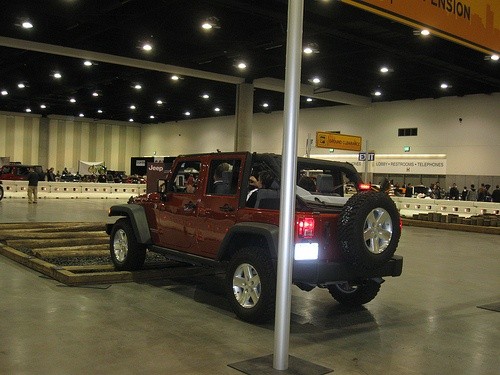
left=186, top=164, right=236, bottom=196
left=46, top=167, right=149, bottom=182
left=377, top=177, right=500, bottom=203
left=26, top=167, right=39, bottom=203
left=246, top=170, right=277, bottom=207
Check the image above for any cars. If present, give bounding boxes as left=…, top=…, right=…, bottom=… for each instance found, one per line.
left=371, top=185, right=381, bottom=192
left=58, top=170, right=142, bottom=183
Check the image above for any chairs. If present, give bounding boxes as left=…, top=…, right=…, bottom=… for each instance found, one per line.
left=245, top=174, right=342, bottom=209
left=214, top=172, right=234, bottom=194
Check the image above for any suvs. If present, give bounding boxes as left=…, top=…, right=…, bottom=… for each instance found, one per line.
left=0, top=164, right=45, bottom=181
left=105, top=152, right=404, bottom=325
